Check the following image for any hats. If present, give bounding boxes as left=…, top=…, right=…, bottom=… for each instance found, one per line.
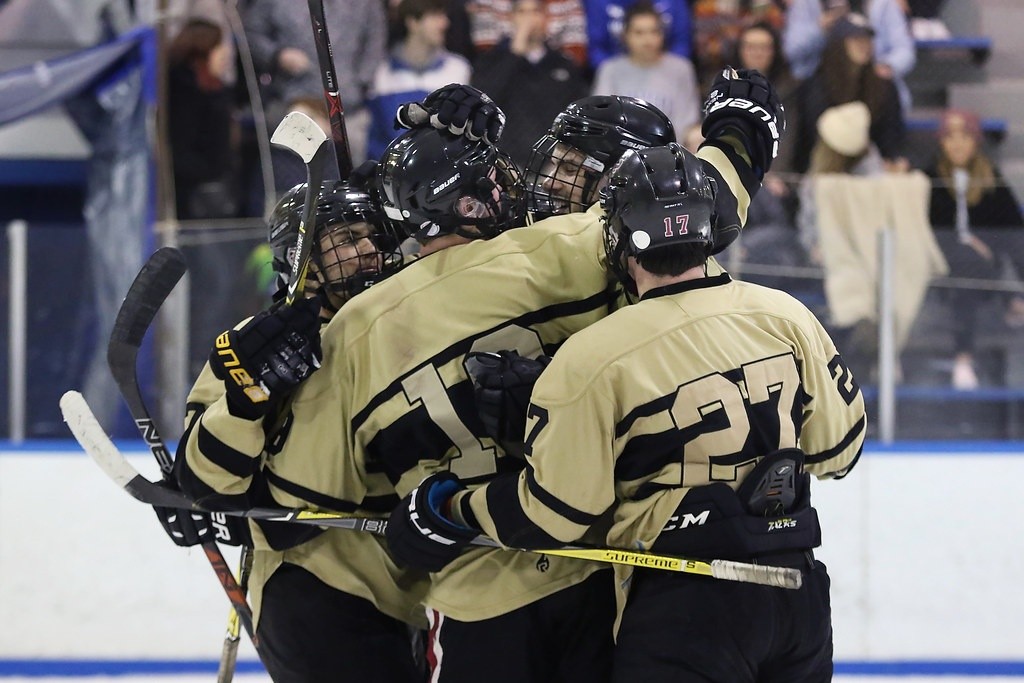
left=937, top=111, right=983, bottom=139
left=817, top=100, right=870, bottom=156
left=832, top=13, right=876, bottom=39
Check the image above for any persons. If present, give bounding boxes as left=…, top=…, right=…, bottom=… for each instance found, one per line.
left=170, top=66, right=867, bottom=683
left=164, top=0, right=1023, bottom=392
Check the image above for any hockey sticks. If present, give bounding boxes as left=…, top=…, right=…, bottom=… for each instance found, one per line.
left=102, top=244, right=269, bottom=672
left=215, top=104, right=334, bottom=683
left=53, top=383, right=807, bottom=593
left=303, top=0, right=355, bottom=183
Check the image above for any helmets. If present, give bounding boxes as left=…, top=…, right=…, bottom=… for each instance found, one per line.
left=267, top=177, right=404, bottom=302
left=377, top=126, right=527, bottom=238
left=599, top=143, right=718, bottom=296
left=521, top=94, right=676, bottom=219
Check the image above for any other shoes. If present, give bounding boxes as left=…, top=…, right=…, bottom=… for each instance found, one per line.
left=952, top=357, right=977, bottom=391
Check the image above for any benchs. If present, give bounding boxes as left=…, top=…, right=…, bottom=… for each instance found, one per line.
left=791, top=35, right=1024, bottom=437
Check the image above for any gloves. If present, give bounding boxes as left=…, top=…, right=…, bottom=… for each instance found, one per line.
left=152, top=480, right=242, bottom=546
left=702, top=63, right=786, bottom=172
left=469, top=349, right=554, bottom=442
left=208, top=296, right=322, bottom=421
left=394, top=82, right=506, bottom=145
left=385, top=470, right=480, bottom=573
